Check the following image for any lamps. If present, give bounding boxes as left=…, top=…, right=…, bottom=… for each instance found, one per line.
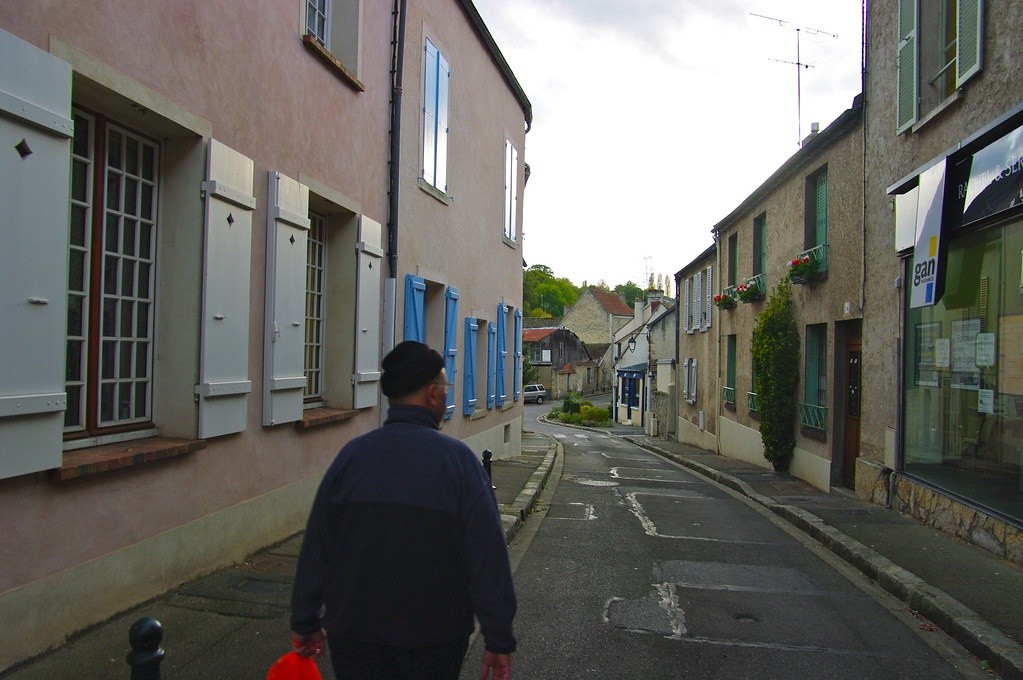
left=628, top=334, right=635, bottom=353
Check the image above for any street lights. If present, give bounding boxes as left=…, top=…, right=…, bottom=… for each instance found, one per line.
left=627, top=332, right=651, bottom=411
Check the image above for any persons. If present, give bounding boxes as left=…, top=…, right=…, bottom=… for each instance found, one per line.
left=289, top=340, right=518, bottom=680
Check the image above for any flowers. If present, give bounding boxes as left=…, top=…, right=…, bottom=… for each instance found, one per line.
left=714, top=295, right=734, bottom=309
left=734, top=281, right=760, bottom=301
left=785, top=256, right=816, bottom=278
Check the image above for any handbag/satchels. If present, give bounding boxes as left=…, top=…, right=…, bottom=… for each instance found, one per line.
left=265, top=636, right=323, bottom=680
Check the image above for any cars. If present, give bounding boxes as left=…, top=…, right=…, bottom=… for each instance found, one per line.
left=523, top=383, right=547, bottom=404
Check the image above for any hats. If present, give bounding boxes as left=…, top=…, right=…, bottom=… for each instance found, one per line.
left=379, top=342, right=447, bottom=398
